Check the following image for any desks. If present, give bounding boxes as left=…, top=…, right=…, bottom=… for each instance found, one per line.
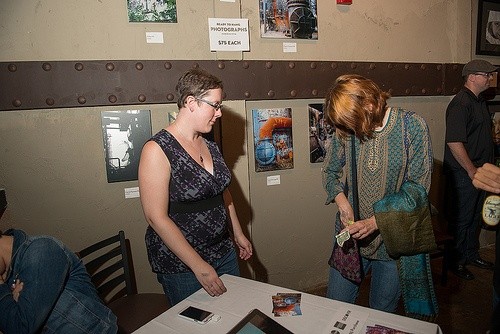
left=129, top=273, right=442, bottom=334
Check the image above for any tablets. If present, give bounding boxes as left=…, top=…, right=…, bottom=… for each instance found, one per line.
left=227, top=308, right=294, bottom=334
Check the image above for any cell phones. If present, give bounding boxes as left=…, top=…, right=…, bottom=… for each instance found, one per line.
left=178, top=306, right=214, bottom=325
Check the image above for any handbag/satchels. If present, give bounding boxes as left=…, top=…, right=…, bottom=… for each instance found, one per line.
left=328, top=219, right=362, bottom=286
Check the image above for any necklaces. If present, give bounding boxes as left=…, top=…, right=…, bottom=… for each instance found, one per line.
left=199, top=146, right=203, bottom=162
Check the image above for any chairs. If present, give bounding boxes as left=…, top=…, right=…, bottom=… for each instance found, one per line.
left=74, top=230, right=172, bottom=334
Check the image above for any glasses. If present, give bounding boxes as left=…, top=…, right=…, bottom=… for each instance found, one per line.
left=474, top=73, right=493, bottom=78
left=201, top=100, right=223, bottom=111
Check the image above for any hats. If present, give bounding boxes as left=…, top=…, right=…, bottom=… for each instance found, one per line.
left=462, top=59, right=499, bottom=77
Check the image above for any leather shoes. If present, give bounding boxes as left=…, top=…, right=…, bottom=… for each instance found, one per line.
left=453, top=264, right=475, bottom=279
left=473, top=257, right=493, bottom=269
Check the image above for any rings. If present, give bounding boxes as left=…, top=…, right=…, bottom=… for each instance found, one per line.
left=208, top=285, right=213, bottom=288
left=358, top=232, right=362, bottom=236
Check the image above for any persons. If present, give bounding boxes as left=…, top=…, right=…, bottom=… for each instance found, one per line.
left=438, top=60, right=500, bottom=280
left=0, top=228, right=125, bottom=334
left=321, top=74, right=434, bottom=313
left=138, top=68, right=252, bottom=307
